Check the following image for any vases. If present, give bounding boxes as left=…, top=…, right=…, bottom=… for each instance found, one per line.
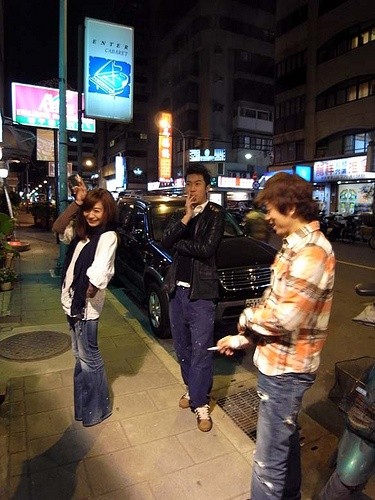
left=0, top=281, right=12, bottom=291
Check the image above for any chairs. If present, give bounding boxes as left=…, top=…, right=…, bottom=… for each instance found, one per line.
left=150, top=217, right=164, bottom=240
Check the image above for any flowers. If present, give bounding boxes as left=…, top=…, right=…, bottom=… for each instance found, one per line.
left=0, top=266, right=17, bottom=284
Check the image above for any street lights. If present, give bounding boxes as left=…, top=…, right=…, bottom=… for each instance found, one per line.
left=156, top=113, right=186, bottom=179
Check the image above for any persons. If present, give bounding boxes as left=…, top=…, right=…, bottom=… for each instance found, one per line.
left=217, top=172, right=336, bottom=500
left=161, top=166, right=225, bottom=432
left=52, top=177, right=119, bottom=427
left=245, top=201, right=271, bottom=243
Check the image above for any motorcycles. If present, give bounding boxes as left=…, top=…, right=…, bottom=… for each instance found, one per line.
left=223, top=200, right=374, bottom=245
left=309, top=355, right=375, bottom=500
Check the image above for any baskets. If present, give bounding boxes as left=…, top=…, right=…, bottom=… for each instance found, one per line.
left=327, top=355, right=375, bottom=431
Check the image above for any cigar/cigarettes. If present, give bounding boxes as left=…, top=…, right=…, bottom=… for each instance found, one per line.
left=207, top=346, right=220, bottom=351
left=77, top=173, right=81, bottom=183
left=183, top=194, right=189, bottom=197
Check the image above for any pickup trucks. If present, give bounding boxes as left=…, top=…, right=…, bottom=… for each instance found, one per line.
left=116, top=195, right=279, bottom=339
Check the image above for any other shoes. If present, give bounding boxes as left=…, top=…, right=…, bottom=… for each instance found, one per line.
left=193, top=404, right=212, bottom=432
left=179, top=390, right=192, bottom=409
